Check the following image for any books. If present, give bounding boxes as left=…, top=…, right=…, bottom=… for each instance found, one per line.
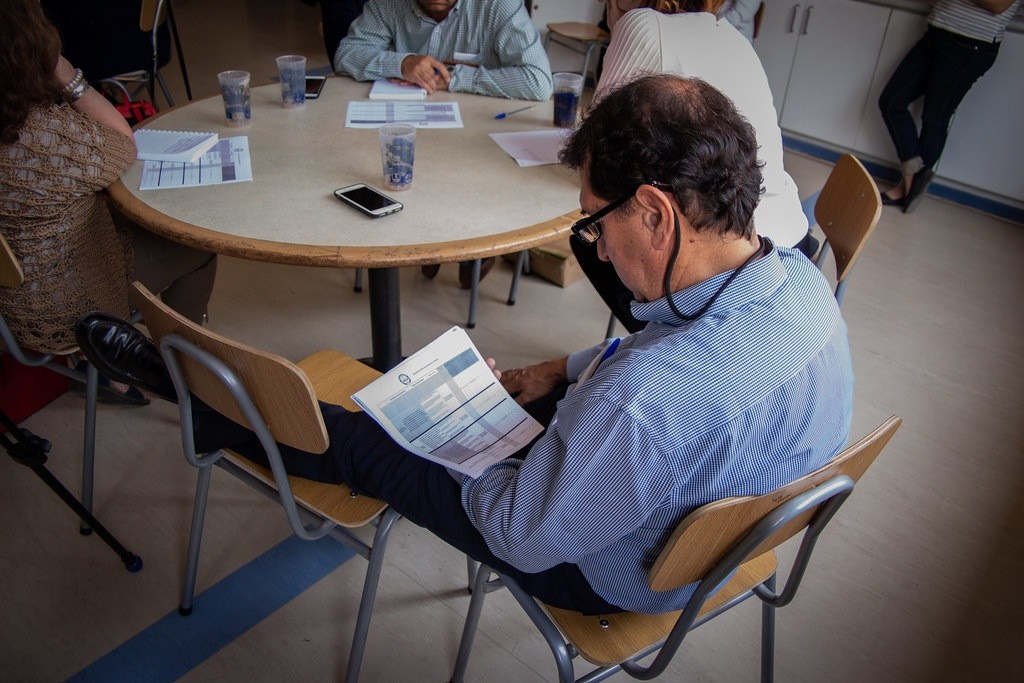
left=369, top=80, right=427, bottom=101
left=133, top=129, right=219, bottom=162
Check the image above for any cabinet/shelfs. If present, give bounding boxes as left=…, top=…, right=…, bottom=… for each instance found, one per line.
left=753, top=0, right=1024, bottom=223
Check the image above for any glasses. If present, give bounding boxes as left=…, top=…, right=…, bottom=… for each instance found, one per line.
left=571, top=177, right=660, bottom=247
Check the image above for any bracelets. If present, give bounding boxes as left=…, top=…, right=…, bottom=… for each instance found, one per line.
left=63, top=69, right=89, bottom=103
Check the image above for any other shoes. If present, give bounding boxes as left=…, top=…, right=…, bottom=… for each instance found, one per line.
left=421, top=263, right=440, bottom=279
left=902, top=167, right=935, bottom=214
left=881, top=190, right=909, bottom=205
left=459, top=257, right=495, bottom=289
left=68, top=362, right=151, bottom=406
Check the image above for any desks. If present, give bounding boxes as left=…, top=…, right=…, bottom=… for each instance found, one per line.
left=105, top=73, right=591, bottom=378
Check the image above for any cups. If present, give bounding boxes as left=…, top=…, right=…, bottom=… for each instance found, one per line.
left=218, top=70, right=251, bottom=127
left=276, top=55, right=307, bottom=109
left=378, top=122, right=416, bottom=191
left=552, top=72, right=584, bottom=129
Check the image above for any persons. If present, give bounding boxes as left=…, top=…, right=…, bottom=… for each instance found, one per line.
left=334, top=0, right=554, bottom=288
left=594, top=0, right=809, bottom=248
left=0, top=0, right=218, bottom=405
left=879, top=0, right=1024, bottom=214
left=75, top=73, right=853, bottom=616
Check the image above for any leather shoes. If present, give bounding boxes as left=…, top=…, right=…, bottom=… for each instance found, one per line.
left=75, top=310, right=180, bottom=406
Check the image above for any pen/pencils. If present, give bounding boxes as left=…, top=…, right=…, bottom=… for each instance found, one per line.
left=590, top=338, right=620, bottom=378
left=495, top=105, right=533, bottom=120
left=435, top=68, right=440, bottom=75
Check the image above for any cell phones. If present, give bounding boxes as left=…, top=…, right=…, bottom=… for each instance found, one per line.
left=334, top=182, right=404, bottom=219
left=305, top=75, right=326, bottom=99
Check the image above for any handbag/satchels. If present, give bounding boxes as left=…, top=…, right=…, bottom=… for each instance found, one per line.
left=101, top=83, right=156, bottom=127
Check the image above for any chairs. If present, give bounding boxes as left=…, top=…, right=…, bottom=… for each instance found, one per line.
left=0, top=0, right=903, bottom=683
